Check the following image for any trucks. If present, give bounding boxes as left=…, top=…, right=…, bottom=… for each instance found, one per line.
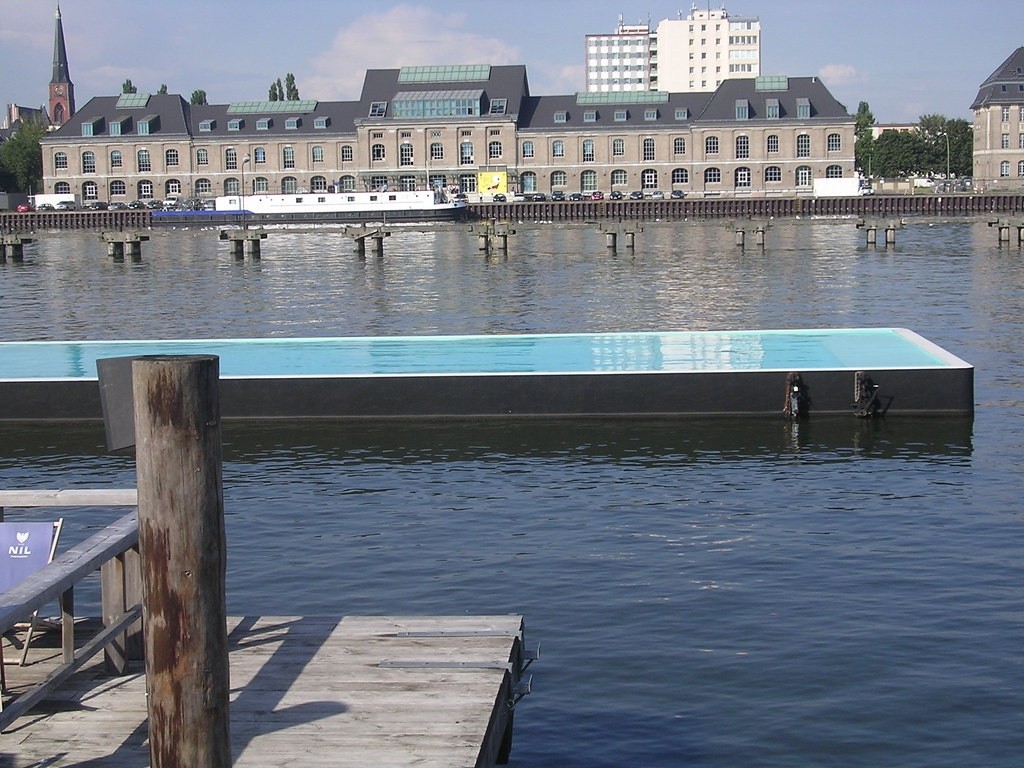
left=163, top=193, right=183, bottom=206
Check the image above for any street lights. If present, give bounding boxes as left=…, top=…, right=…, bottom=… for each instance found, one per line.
left=242, top=156, right=250, bottom=228
left=944, top=133, right=950, bottom=180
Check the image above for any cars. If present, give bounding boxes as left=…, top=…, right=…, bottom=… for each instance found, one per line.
left=89, top=201, right=106, bottom=211
left=18, top=204, right=33, bottom=212
left=493, top=194, right=506, bottom=202
left=551, top=191, right=566, bottom=202
left=630, top=191, right=644, bottom=200
left=128, top=200, right=145, bottom=209
left=449, top=192, right=469, bottom=204
left=569, top=193, right=583, bottom=201
left=108, top=202, right=127, bottom=211
left=532, top=193, right=547, bottom=202
left=609, top=191, right=623, bottom=200
left=671, top=190, right=684, bottom=199
left=652, top=191, right=665, bottom=199
left=55, top=201, right=77, bottom=211
left=591, top=192, right=604, bottom=200
left=147, top=199, right=162, bottom=209
left=861, top=185, right=874, bottom=196
left=37, top=203, right=53, bottom=211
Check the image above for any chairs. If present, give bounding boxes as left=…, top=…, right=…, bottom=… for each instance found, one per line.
left=0, top=518, right=63, bottom=667
